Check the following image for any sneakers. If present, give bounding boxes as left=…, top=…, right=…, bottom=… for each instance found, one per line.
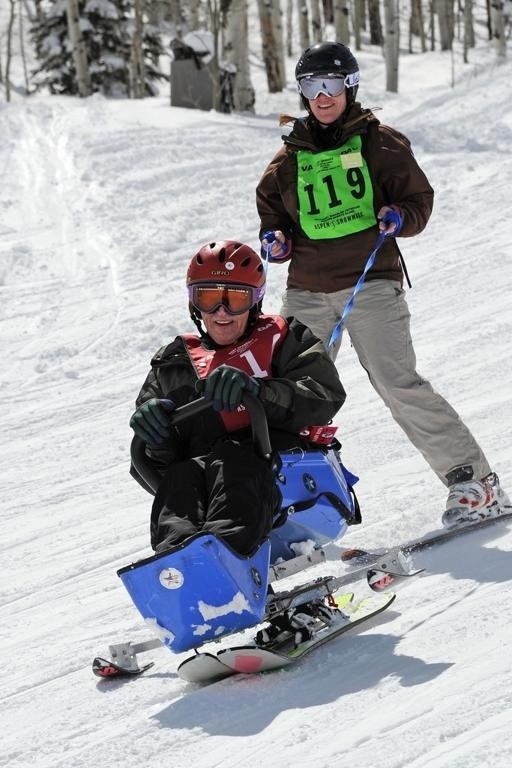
left=443, top=474, right=509, bottom=525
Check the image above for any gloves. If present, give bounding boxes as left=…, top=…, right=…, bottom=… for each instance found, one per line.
left=194, top=365, right=262, bottom=413
left=131, top=399, right=176, bottom=448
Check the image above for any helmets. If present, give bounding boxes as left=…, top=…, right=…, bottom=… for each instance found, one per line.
left=295, top=42, right=359, bottom=105
left=188, top=240, right=266, bottom=319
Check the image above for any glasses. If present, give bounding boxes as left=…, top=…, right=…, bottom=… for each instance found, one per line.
left=299, top=74, right=348, bottom=100
left=188, top=285, right=257, bottom=315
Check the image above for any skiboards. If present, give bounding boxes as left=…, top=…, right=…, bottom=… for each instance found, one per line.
left=175, top=588, right=398, bottom=683
left=341, top=507, right=512, bottom=569
left=93, top=656, right=155, bottom=678
left=94, top=509, right=512, bottom=678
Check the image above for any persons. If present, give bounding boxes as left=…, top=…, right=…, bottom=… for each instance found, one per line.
left=132, top=240, right=347, bottom=561
left=254, top=41, right=512, bottom=531
